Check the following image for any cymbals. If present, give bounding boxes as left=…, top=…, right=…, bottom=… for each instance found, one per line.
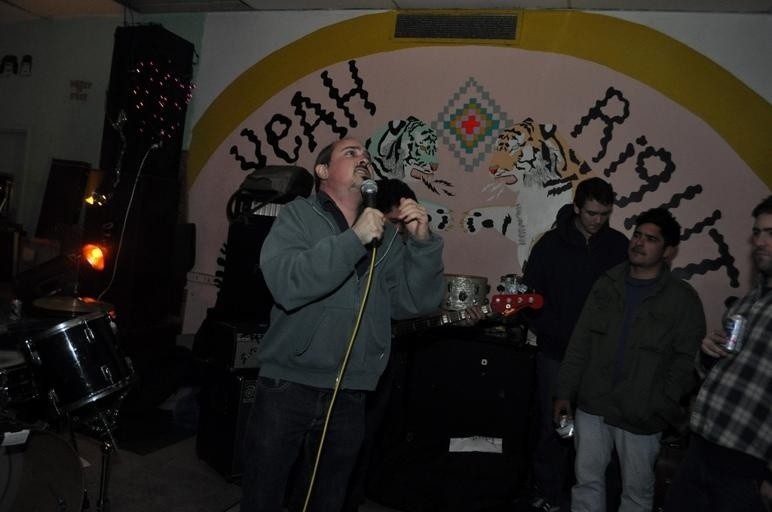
left=33, top=296, right=115, bottom=313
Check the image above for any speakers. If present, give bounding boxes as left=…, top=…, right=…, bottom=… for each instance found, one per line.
left=98, top=21, right=195, bottom=176
left=79, top=175, right=185, bottom=355
left=34, top=157, right=93, bottom=240
left=213, top=164, right=315, bottom=324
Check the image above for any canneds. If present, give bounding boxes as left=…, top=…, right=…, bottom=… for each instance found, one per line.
left=721, top=314, right=749, bottom=354
left=8, top=299, right=23, bottom=320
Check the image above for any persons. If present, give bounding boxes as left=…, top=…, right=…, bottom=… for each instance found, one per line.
left=242, top=140, right=446, bottom=512
left=519, top=178, right=631, bottom=512
left=552, top=206, right=707, bottom=512
left=687, top=193, right=772, bottom=512
left=363, top=178, right=486, bottom=512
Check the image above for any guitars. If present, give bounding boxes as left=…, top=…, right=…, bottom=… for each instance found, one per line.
left=391, top=284, right=543, bottom=336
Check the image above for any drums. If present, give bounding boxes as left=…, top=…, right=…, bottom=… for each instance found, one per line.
left=500, top=275, right=523, bottom=296
left=23, top=309, right=133, bottom=418
left=441, top=272, right=490, bottom=311
left=0, top=351, right=46, bottom=437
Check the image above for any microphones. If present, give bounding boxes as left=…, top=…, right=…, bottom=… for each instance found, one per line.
left=360, top=179, right=380, bottom=248
